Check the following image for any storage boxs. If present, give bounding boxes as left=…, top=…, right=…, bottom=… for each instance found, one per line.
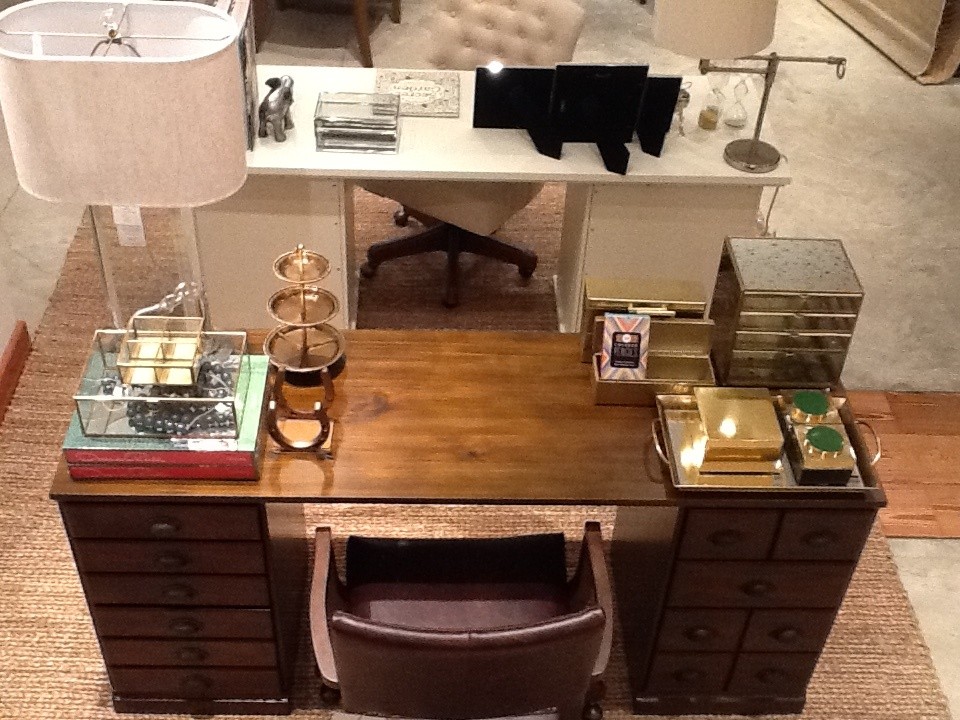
left=62, top=316, right=271, bottom=482
left=593, top=236, right=866, bottom=406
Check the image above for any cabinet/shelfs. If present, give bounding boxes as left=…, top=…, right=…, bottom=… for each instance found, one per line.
left=264, top=242, right=346, bottom=388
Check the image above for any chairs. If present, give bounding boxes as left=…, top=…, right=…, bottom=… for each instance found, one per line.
left=352, top=0, right=586, bottom=309
left=310, top=520, right=615, bottom=720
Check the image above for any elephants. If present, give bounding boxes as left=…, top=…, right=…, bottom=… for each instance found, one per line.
left=258, top=75, right=295, bottom=142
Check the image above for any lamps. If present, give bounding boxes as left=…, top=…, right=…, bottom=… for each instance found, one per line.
left=652, top=1, right=846, bottom=173
left=0, top=0, right=248, bottom=328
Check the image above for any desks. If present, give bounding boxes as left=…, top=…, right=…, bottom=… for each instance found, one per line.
left=47, top=332, right=888, bottom=715
left=183, top=65, right=794, bottom=333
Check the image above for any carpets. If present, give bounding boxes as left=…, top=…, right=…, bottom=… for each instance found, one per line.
left=0, top=181, right=960, bottom=720
